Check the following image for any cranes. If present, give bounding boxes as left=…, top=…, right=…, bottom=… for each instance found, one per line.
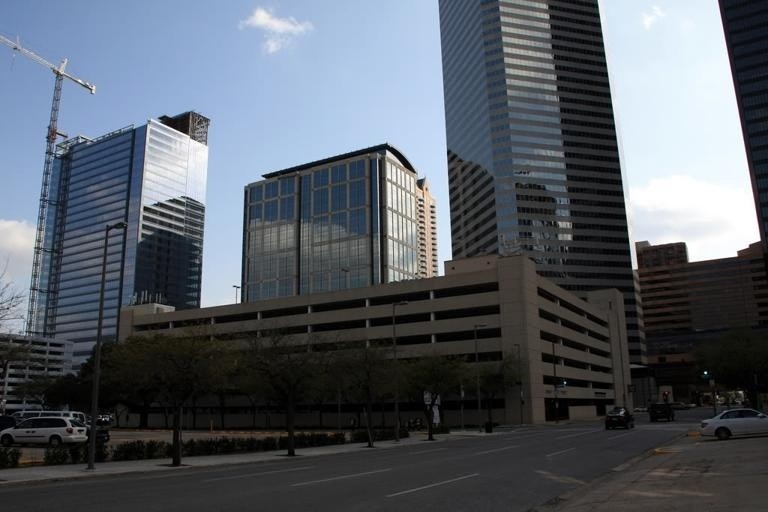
left=0, top=32, right=96, bottom=338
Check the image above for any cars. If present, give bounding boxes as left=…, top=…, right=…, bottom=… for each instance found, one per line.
left=700, top=407, right=767, bottom=439
left=0, top=406, right=112, bottom=449
left=603, top=399, right=690, bottom=430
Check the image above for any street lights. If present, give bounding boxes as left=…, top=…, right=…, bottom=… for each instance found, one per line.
left=340, top=267, right=349, bottom=289
left=78, top=220, right=128, bottom=471
left=386, top=299, right=523, bottom=442
left=232, top=284, right=240, bottom=304
